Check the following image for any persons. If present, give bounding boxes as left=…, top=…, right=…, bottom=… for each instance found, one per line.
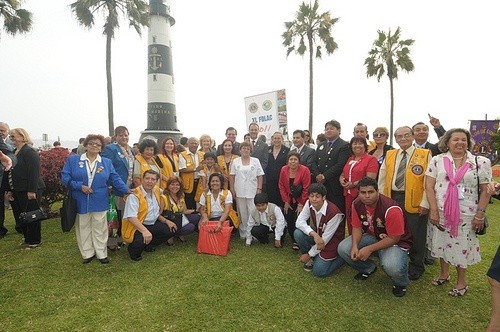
left=486, top=179, right=500, bottom=332
left=373, top=126, right=431, bottom=279
left=60, top=132, right=132, bottom=263
left=335, top=179, right=411, bottom=297
left=425, top=129, right=494, bottom=296
left=75, top=121, right=452, bottom=244
left=117, top=169, right=176, bottom=262
left=0, top=121, right=46, bottom=246
left=412, top=113, right=447, bottom=157
left=291, top=183, right=347, bottom=278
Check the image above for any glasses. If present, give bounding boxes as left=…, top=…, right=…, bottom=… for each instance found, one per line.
left=374, top=134, right=385, bottom=138
left=87, top=141, right=101, bottom=146
left=396, top=133, right=412, bottom=140
left=8, top=135, right=14, bottom=139
left=165, top=143, right=174, bottom=145
left=305, top=135, right=310, bottom=137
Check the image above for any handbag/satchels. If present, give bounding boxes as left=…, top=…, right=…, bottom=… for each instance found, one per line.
left=108, top=193, right=118, bottom=238
left=162, top=210, right=182, bottom=229
left=19, top=198, right=45, bottom=224
left=475, top=217, right=489, bottom=235
left=60, top=199, right=77, bottom=232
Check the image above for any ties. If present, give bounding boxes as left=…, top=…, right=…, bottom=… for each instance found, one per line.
left=395, top=150, right=407, bottom=189
left=252, top=139, right=255, bottom=148
left=326, top=141, right=332, bottom=152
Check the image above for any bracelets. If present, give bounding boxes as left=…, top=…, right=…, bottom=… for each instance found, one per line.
left=473, top=206, right=487, bottom=220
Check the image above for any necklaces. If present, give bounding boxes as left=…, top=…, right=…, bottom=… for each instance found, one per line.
left=453, top=156, right=463, bottom=160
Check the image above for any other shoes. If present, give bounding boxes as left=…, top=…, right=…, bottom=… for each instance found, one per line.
left=167, top=236, right=186, bottom=246
left=83, top=255, right=109, bottom=264
left=431, top=275, right=468, bottom=297
left=303, top=257, right=314, bottom=272
left=392, top=284, right=406, bottom=297
left=424, top=258, right=434, bottom=264
left=17, top=241, right=42, bottom=249
left=408, top=268, right=425, bottom=280
left=131, top=244, right=156, bottom=261
left=292, top=242, right=300, bottom=250
left=354, top=267, right=377, bottom=280
